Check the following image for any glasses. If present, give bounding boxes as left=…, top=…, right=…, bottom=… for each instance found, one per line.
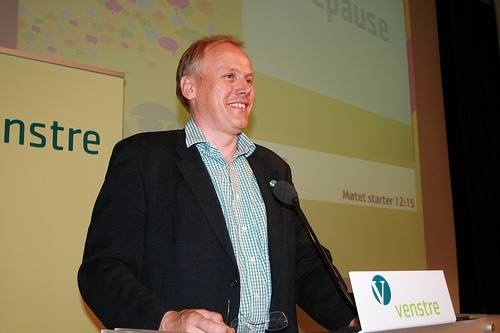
left=231, top=311, right=288, bottom=333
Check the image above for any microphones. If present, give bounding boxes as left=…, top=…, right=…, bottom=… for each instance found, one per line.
left=273, top=181, right=357, bottom=311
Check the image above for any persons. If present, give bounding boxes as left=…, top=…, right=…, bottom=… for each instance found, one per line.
left=78, top=33, right=359, bottom=333
